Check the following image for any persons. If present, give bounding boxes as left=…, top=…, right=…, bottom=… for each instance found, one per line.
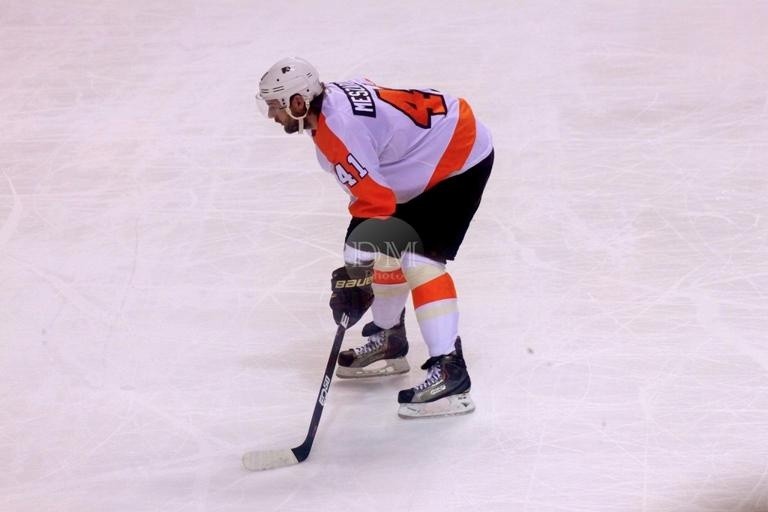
left=255, top=55, right=495, bottom=418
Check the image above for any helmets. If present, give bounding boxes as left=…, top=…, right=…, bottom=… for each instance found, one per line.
left=255, top=56, right=324, bottom=119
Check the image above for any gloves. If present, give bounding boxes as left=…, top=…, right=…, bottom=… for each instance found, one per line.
left=328, top=267, right=374, bottom=329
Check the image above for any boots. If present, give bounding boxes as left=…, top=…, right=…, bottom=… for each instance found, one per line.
left=338, top=307, right=407, bottom=367
left=397, top=335, right=470, bottom=403
